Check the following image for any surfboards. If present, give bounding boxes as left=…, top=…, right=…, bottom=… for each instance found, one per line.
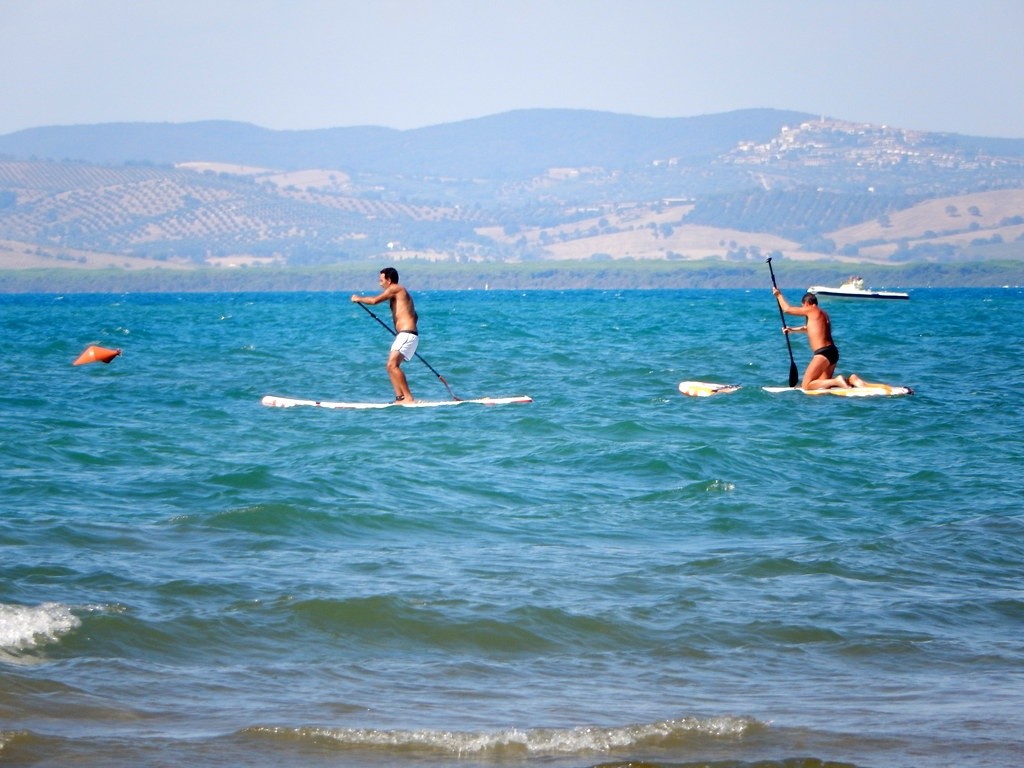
left=262, top=394, right=535, bottom=408
left=677, top=380, right=914, bottom=397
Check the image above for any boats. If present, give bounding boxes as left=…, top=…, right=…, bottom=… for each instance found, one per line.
left=806, top=275, right=910, bottom=300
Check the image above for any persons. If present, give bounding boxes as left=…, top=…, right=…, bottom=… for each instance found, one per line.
left=773, top=288, right=866, bottom=391
left=352, top=268, right=419, bottom=405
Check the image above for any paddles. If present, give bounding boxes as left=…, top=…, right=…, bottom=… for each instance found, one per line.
left=765, top=256, right=806, bottom=387
left=357, top=301, right=462, bottom=400
